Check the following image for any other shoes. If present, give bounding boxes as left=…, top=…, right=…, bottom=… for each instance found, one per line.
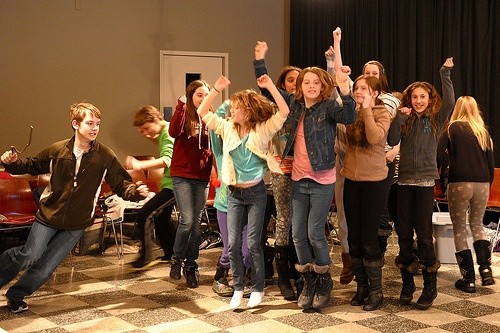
left=263, top=278, right=274, bottom=289
left=126, top=256, right=161, bottom=270
left=155, top=254, right=173, bottom=262
left=212, top=280, right=234, bottom=296
left=242, top=282, right=252, bottom=296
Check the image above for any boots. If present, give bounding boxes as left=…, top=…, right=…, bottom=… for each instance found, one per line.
left=394, top=253, right=420, bottom=301
left=276, top=264, right=295, bottom=300
left=312, top=262, right=334, bottom=308
left=377, top=227, right=394, bottom=268
left=415, top=258, right=441, bottom=308
left=363, top=253, right=386, bottom=310
left=291, top=266, right=304, bottom=295
left=339, top=251, right=355, bottom=284
left=473, top=239, right=496, bottom=286
left=454, top=249, right=476, bottom=293
left=350, top=257, right=370, bottom=305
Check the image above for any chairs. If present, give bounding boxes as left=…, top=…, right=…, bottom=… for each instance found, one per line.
left=0, top=155, right=222, bottom=267
left=429, top=167, right=500, bottom=254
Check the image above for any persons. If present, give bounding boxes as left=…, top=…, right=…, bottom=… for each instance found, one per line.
left=0, top=97, right=149, bottom=312
left=436, top=95, right=495, bottom=293
left=197, top=26, right=402, bottom=313
left=387, top=57, right=456, bottom=313
left=124, top=105, right=177, bottom=271
left=168, top=76, right=215, bottom=288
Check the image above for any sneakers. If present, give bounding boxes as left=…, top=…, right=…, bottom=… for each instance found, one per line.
left=7, top=297, right=29, bottom=313
left=294, top=261, right=318, bottom=308
left=168, top=258, right=184, bottom=279
left=182, top=268, right=200, bottom=288
